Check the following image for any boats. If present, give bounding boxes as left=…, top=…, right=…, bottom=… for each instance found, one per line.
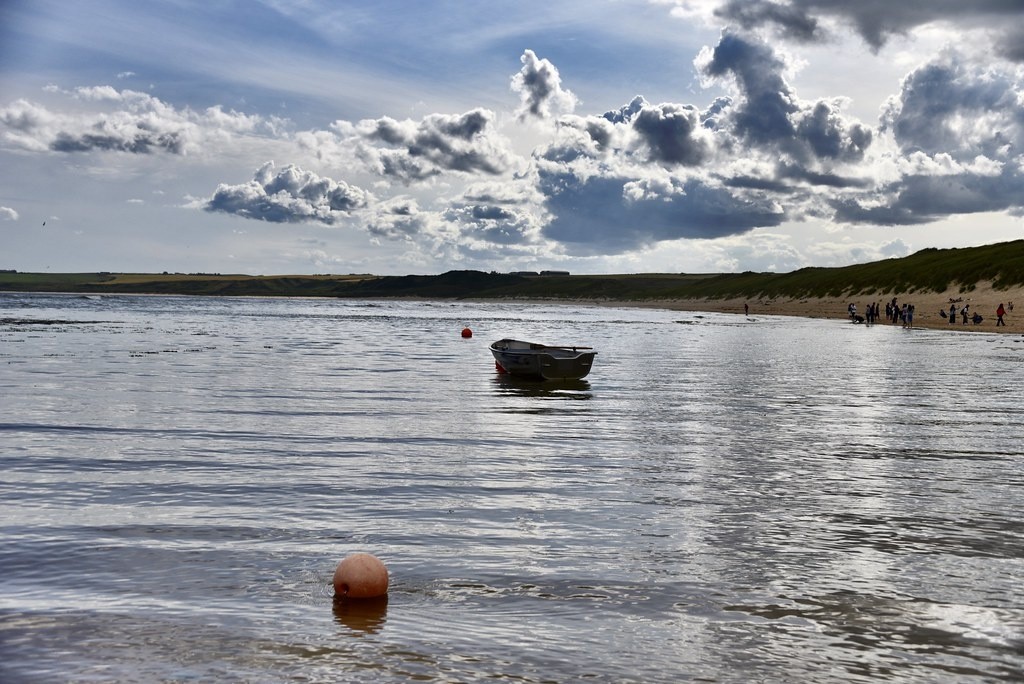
left=488, top=338, right=599, bottom=382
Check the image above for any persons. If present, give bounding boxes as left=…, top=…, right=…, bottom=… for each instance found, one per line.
left=745, top=303, right=748, bottom=315
left=848, top=297, right=913, bottom=329
left=996, top=302, right=1013, bottom=326
left=949, top=304, right=955, bottom=325
left=962, top=304, right=983, bottom=324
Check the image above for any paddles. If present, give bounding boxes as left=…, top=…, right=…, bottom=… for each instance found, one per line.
left=529, top=343, right=593, bottom=351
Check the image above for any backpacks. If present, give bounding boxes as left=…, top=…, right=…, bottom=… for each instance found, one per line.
left=961, top=307, right=968, bottom=315
